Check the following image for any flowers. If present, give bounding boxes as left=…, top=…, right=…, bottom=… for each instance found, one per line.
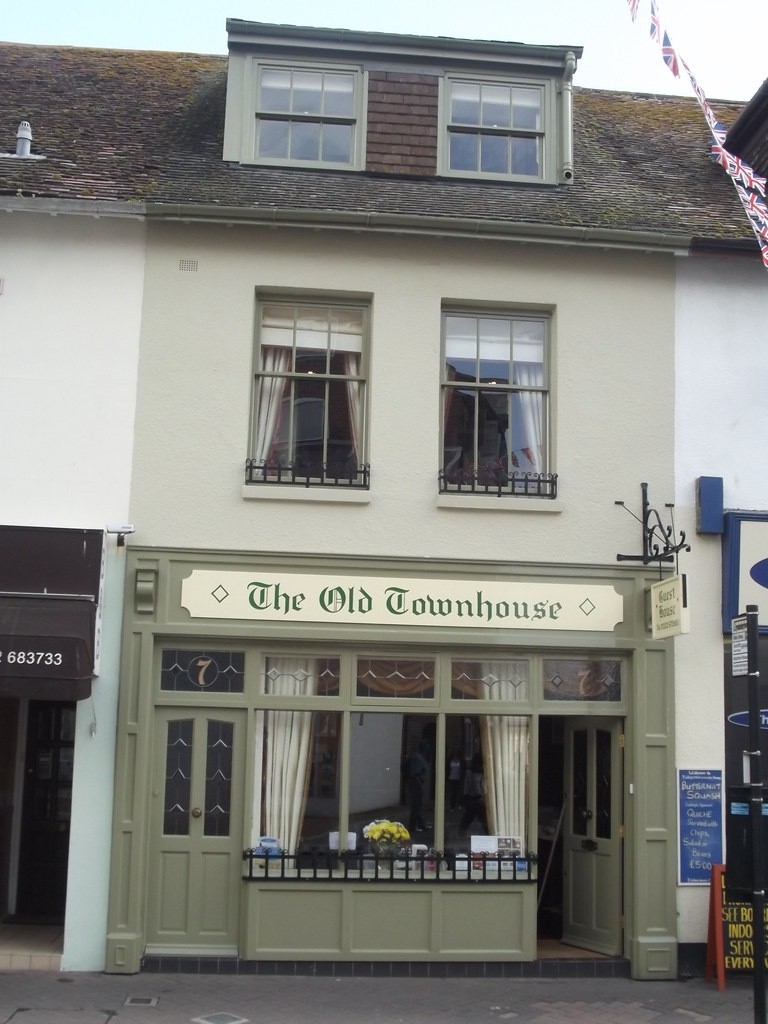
left=363, top=819, right=412, bottom=859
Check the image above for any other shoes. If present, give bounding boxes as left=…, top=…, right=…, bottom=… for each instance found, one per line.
left=414, top=824, right=432, bottom=833
left=455, top=832, right=471, bottom=840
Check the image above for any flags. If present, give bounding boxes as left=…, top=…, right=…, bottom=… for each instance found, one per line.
left=630, top=0, right=767, bottom=268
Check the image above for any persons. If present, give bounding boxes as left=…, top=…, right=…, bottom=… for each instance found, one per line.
left=447, top=749, right=465, bottom=810
left=458, top=753, right=489, bottom=839
left=404, top=741, right=432, bottom=833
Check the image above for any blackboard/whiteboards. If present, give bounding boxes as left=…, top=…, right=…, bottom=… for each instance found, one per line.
left=713, top=864, right=768, bottom=970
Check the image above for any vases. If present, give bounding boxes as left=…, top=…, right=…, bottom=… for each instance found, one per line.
left=377, top=854, right=395, bottom=875
